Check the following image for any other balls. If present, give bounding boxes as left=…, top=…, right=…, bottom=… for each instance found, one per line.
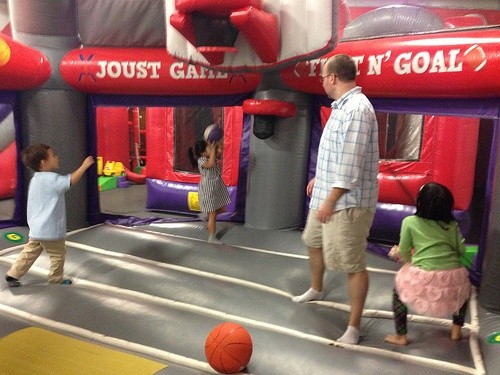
left=203, top=123, right=223, bottom=145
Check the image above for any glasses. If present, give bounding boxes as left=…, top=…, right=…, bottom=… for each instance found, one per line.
left=321, top=73, right=338, bottom=83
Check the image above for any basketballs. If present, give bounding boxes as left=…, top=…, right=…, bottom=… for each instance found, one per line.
left=202, top=322, right=254, bottom=374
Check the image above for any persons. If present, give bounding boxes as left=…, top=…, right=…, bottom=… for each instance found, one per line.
left=291, top=55, right=380, bottom=344
left=383, top=182, right=472, bottom=346
left=5, top=143, right=94, bottom=287
left=188, top=138, right=231, bottom=245
